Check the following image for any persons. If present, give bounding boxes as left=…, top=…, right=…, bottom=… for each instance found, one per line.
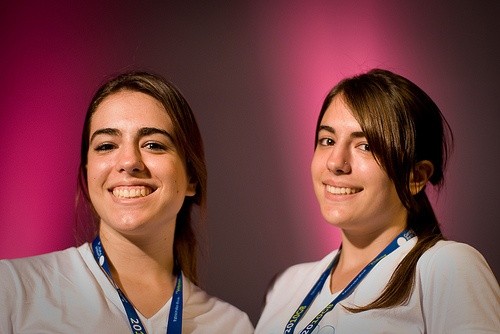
left=251, top=68, right=500, bottom=334
left=1, top=71, right=254, bottom=333
